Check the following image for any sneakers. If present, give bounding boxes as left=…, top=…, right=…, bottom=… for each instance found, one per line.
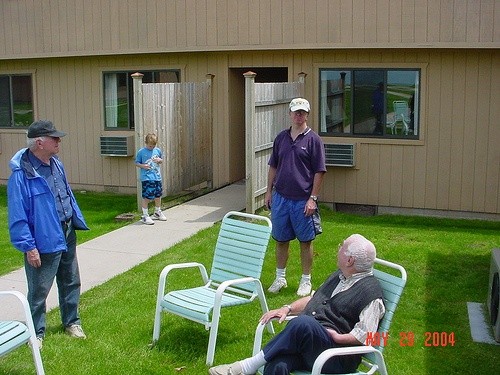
left=154, top=209, right=167, bottom=221
left=268, top=278, right=287, bottom=293
left=65, top=325, right=86, bottom=339
left=140, top=214, right=154, bottom=225
left=297, top=279, right=312, bottom=296
left=28, top=336, right=42, bottom=350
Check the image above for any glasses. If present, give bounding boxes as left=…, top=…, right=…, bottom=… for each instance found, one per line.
left=338, top=243, right=356, bottom=262
left=291, top=109, right=309, bottom=115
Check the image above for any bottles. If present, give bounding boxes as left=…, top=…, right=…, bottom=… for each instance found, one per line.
left=145, top=160, right=153, bottom=171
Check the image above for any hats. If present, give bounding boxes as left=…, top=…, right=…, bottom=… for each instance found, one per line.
left=289, top=98, right=310, bottom=112
left=28, top=120, right=65, bottom=138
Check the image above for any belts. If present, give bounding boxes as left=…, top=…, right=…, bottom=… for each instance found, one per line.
left=61, top=217, right=72, bottom=225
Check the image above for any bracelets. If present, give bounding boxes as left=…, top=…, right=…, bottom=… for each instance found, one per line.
left=283, top=305, right=291, bottom=314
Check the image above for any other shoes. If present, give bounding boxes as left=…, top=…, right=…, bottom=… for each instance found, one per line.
left=208, top=361, right=244, bottom=375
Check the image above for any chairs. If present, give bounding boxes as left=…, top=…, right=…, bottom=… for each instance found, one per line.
left=0, top=289, right=46, bottom=375
left=153, top=210, right=276, bottom=366
left=392, top=100, right=410, bottom=136
left=252, top=258, right=408, bottom=375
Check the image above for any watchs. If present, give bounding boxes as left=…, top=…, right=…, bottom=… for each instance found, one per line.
left=310, top=196, right=318, bottom=201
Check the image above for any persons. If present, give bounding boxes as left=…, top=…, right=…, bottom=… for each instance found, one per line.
left=370, top=82, right=383, bottom=133
left=264, top=98, right=326, bottom=296
left=209, top=233, right=385, bottom=375
left=7, top=121, right=90, bottom=350
left=136, top=133, right=166, bottom=225
